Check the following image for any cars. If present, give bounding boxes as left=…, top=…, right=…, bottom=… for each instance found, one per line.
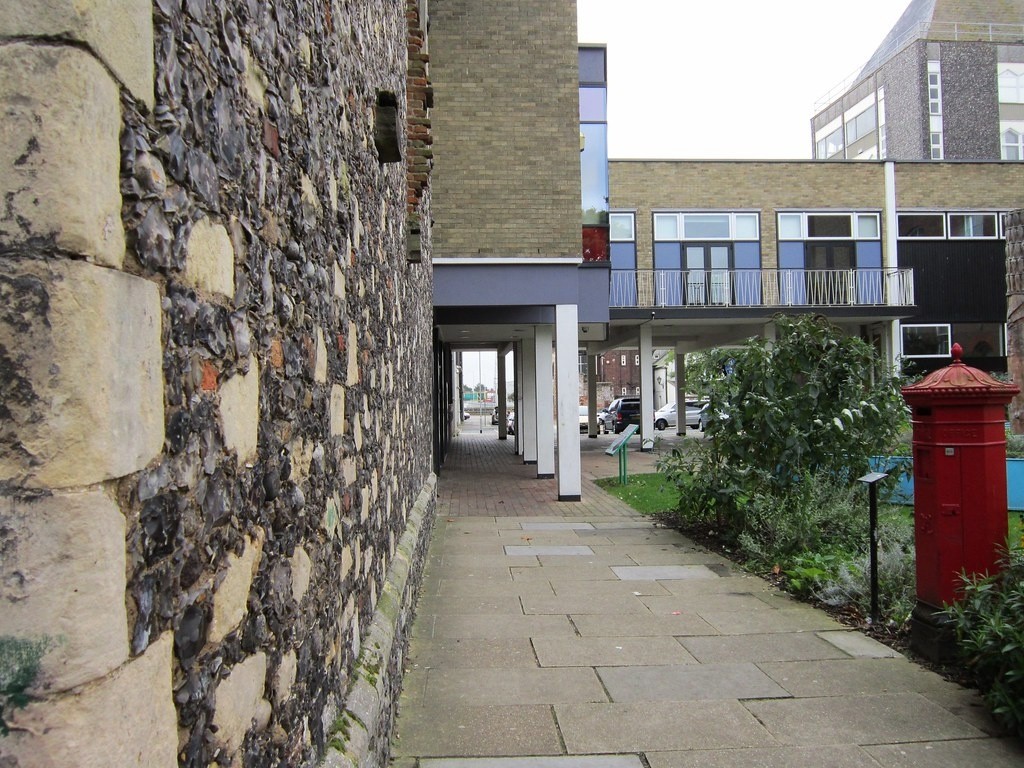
left=464, top=412, right=471, bottom=421
left=698, top=402, right=731, bottom=432
left=490, top=406, right=499, bottom=425
left=579, top=405, right=601, bottom=435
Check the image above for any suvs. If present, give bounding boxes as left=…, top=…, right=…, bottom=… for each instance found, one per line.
left=655, top=395, right=711, bottom=431
left=602, top=397, right=655, bottom=434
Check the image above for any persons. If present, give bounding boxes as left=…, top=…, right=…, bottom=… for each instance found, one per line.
left=506, top=409, right=514, bottom=435
left=492, top=407, right=499, bottom=425
